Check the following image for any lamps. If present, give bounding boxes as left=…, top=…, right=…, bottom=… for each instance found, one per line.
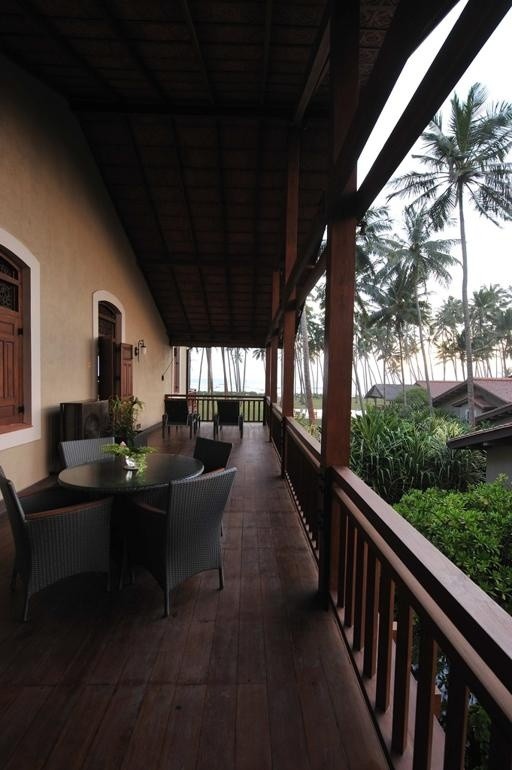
left=135, top=338, right=146, bottom=363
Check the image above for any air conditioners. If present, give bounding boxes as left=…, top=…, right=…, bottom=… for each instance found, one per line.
left=59, top=399, right=109, bottom=466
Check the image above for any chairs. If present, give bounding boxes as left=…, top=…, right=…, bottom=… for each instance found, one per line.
left=0, top=435, right=237, bottom=621
left=162, top=393, right=243, bottom=441
